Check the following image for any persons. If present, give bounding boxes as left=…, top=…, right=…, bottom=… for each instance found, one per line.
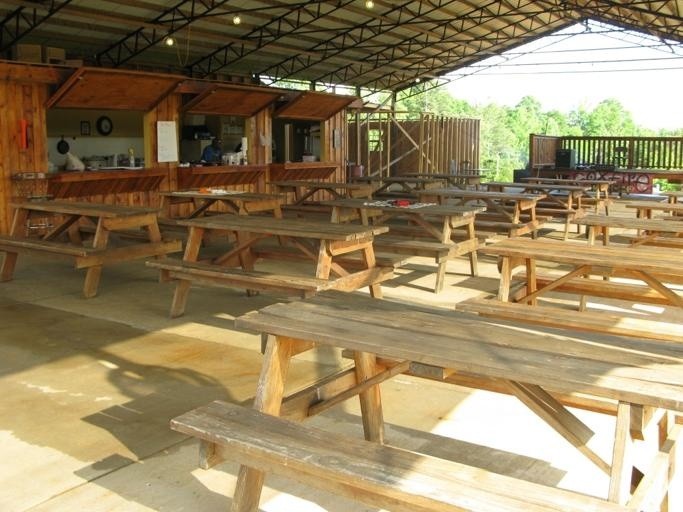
left=199, top=136, right=224, bottom=162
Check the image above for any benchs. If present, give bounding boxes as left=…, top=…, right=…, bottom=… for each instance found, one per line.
left=169, top=400, right=622, bottom=512
left=0, top=234, right=103, bottom=270
left=145, top=255, right=335, bottom=299
left=160, top=173, right=683, bottom=249
left=373, top=237, right=453, bottom=263
left=234, top=241, right=411, bottom=279
left=75, top=224, right=184, bottom=252
left=511, top=269, right=683, bottom=309
left=456, top=297, right=683, bottom=344
left=339, top=348, right=683, bottom=429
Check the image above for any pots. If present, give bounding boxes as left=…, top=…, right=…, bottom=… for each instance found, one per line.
left=57, top=135, right=70, bottom=155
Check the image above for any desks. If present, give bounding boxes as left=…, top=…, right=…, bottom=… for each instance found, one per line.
left=170, top=190, right=683, bottom=512
left=0, top=174, right=617, bottom=317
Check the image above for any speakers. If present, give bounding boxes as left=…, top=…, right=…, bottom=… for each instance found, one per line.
left=555, top=149, right=575, bottom=169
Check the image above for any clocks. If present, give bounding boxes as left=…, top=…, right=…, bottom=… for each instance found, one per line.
left=96, top=115, right=113, bottom=136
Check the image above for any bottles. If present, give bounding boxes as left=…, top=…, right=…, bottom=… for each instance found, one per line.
left=128, top=148, right=135, bottom=167
left=450, top=159, right=456, bottom=175
